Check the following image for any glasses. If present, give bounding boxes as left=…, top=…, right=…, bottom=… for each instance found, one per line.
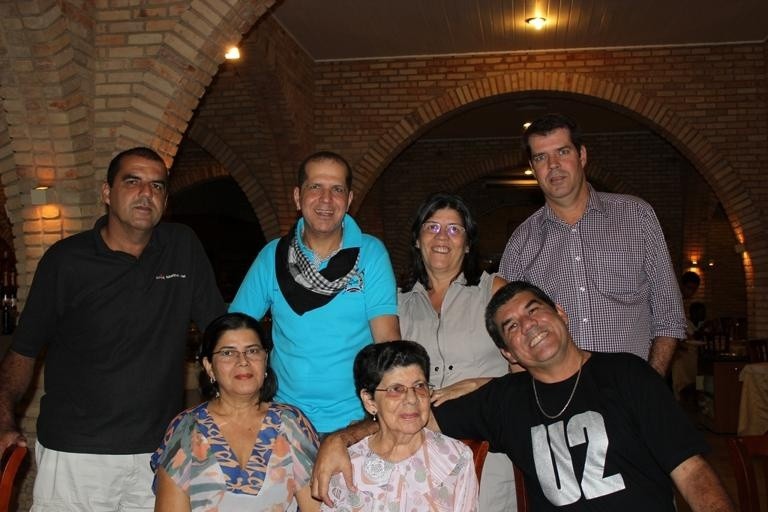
left=209, top=349, right=265, bottom=361
left=421, top=222, right=465, bottom=238
left=375, top=383, right=435, bottom=397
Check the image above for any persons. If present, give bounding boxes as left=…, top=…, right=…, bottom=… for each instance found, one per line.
left=677, top=272, right=701, bottom=300
left=0, top=146, right=227, bottom=512
left=149, top=312, right=322, bottom=512
left=309, top=280, right=736, bottom=512
left=323, top=340, right=480, bottom=512
left=226, top=150, right=402, bottom=440
left=496, top=112, right=692, bottom=375
left=398, top=192, right=510, bottom=410
left=685, top=302, right=711, bottom=354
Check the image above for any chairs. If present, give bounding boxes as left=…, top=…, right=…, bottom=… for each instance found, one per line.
left=511, top=465, right=528, bottom=512
left=688, top=313, right=768, bottom=512
left=459, top=439, right=490, bottom=495
left=0, top=442, right=30, bottom=511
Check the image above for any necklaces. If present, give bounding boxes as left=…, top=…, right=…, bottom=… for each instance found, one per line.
left=532, top=353, right=583, bottom=419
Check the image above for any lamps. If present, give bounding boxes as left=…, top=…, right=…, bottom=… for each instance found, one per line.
left=30, top=182, right=56, bottom=209
left=523, top=15, right=547, bottom=33
